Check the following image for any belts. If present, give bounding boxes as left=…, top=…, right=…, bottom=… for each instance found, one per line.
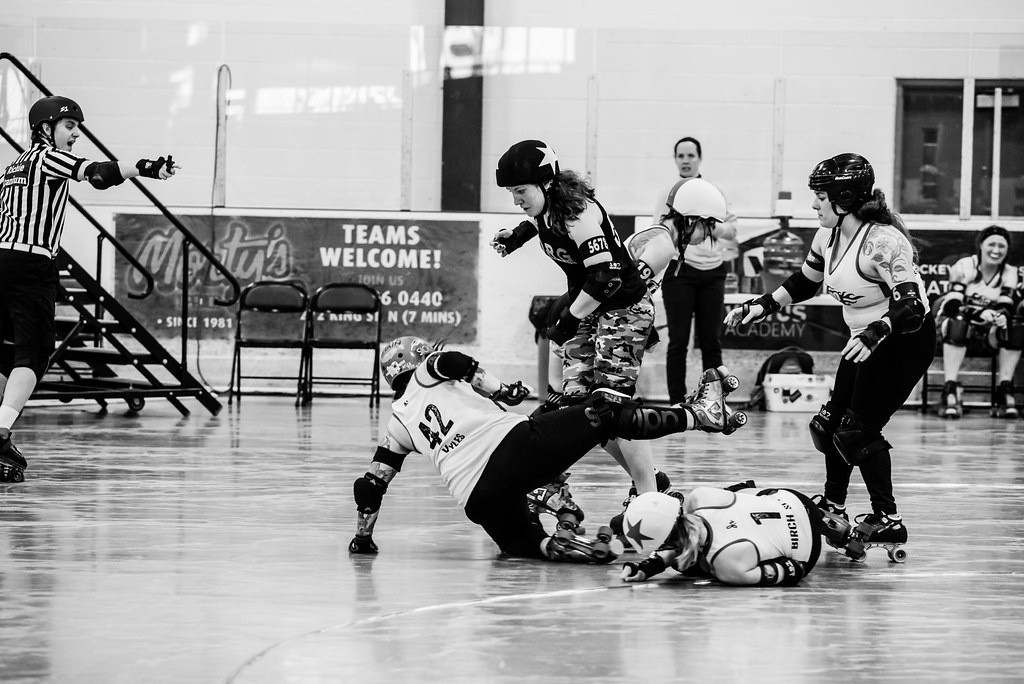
left=0, top=242, right=51, bottom=259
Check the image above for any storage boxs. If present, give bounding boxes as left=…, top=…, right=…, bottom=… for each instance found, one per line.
left=763, top=373, right=830, bottom=413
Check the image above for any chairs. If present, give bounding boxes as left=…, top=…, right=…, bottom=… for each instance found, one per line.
left=303, top=282, right=383, bottom=408
left=227, top=280, right=309, bottom=407
left=921, top=292, right=1024, bottom=416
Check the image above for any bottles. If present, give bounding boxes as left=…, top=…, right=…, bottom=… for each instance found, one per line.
left=762, top=231, right=804, bottom=277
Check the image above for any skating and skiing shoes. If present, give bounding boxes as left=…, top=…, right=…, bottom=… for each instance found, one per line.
left=938, top=380, right=965, bottom=419
left=0, top=432, right=28, bottom=482
left=847, top=510, right=908, bottom=564
left=611, top=510, right=632, bottom=548
left=817, top=506, right=874, bottom=560
left=526, top=472, right=585, bottom=530
left=989, top=379, right=1019, bottom=418
left=623, top=470, right=685, bottom=507
left=546, top=513, right=624, bottom=565
left=811, top=495, right=850, bottom=548
left=679, top=365, right=747, bottom=436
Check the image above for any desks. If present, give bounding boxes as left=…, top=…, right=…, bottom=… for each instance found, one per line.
left=694, top=293, right=852, bottom=353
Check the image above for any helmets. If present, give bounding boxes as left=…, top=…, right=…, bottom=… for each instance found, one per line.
left=808, top=153, right=875, bottom=209
left=666, top=177, right=727, bottom=224
left=29, top=95, right=85, bottom=131
left=495, top=139, right=560, bottom=187
left=380, top=335, right=434, bottom=390
left=623, top=491, right=682, bottom=555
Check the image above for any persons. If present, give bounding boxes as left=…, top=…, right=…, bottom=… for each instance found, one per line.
left=353, top=334, right=727, bottom=565
left=935, top=224, right=1024, bottom=418
left=490, top=139, right=672, bottom=549
left=724, top=154, right=938, bottom=542
left=0, top=96, right=183, bottom=484
left=621, top=487, right=852, bottom=587
left=654, top=137, right=739, bottom=413
